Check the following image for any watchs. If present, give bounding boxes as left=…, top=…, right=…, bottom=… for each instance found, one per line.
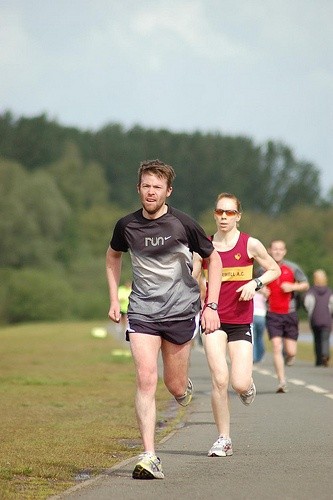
left=205, top=302, right=218, bottom=310
left=255, top=278, right=263, bottom=291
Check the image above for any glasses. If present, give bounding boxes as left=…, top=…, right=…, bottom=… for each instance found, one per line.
left=215, top=209, right=239, bottom=217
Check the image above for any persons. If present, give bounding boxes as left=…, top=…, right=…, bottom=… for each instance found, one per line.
left=105, top=159, right=221, bottom=479
left=191, top=192, right=281, bottom=457
left=116, top=267, right=269, bottom=364
left=254, top=239, right=310, bottom=393
left=303, top=269, right=333, bottom=367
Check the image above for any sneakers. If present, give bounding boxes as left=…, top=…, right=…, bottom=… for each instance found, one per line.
left=238, top=385, right=256, bottom=406
left=316, top=360, right=327, bottom=368
left=176, top=379, right=192, bottom=406
left=133, top=457, right=164, bottom=478
left=277, top=385, right=288, bottom=393
left=286, top=357, right=294, bottom=366
left=208, top=435, right=233, bottom=456
